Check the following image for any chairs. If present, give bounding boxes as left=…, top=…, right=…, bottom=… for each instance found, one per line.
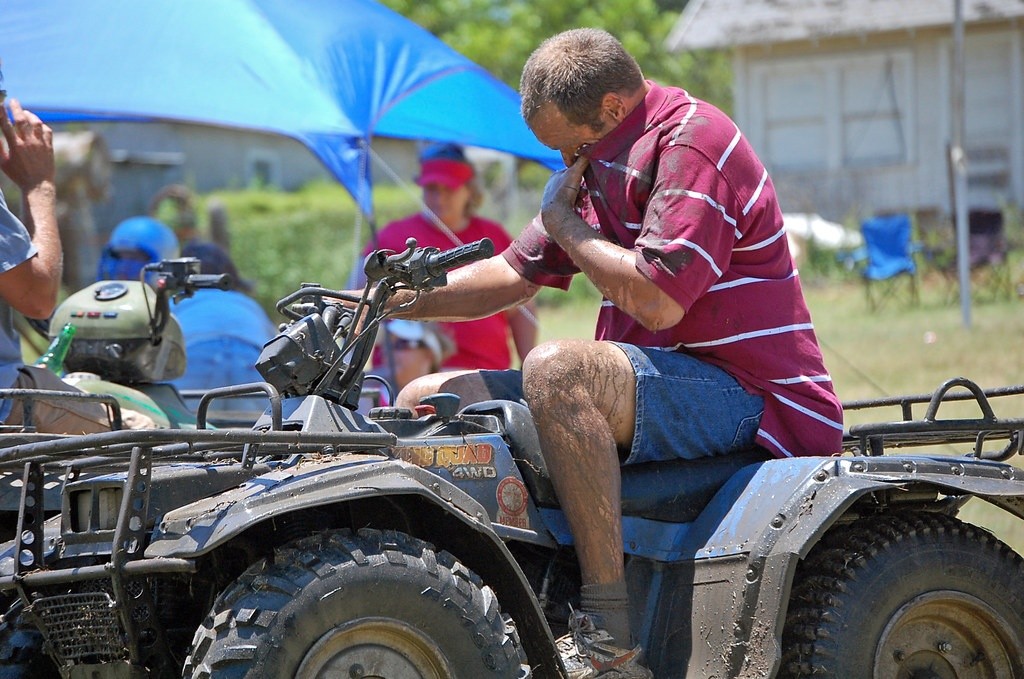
left=160, top=334, right=270, bottom=413
left=834, top=212, right=934, bottom=313
left=929, top=208, right=1013, bottom=308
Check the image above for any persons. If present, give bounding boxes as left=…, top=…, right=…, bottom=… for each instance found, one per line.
left=318, top=27, right=844, bottom=679
left=152, top=242, right=306, bottom=420
left=347, top=140, right=538, bottom=417
left=0, top=71, right=175, bottom=442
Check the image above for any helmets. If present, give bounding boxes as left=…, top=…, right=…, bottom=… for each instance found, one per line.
left=96, top=217, right=182, bottom=288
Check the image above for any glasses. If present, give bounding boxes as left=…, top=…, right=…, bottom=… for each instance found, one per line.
left=392, top=339, right=423, bottom=351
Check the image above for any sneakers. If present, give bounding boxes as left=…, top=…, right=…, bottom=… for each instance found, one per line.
left=555, top=601, right=652, bottom=679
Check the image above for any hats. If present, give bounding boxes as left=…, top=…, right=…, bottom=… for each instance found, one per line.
left=386, top=319, right=443, bottom=369
left=415, top=159, right=474, bottom=192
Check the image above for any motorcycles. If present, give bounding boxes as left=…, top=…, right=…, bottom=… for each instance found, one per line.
left=0, top=239, right=1024, bottom=678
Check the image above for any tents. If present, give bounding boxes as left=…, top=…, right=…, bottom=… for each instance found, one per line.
left=0, top=0, right=570, bottom=408
